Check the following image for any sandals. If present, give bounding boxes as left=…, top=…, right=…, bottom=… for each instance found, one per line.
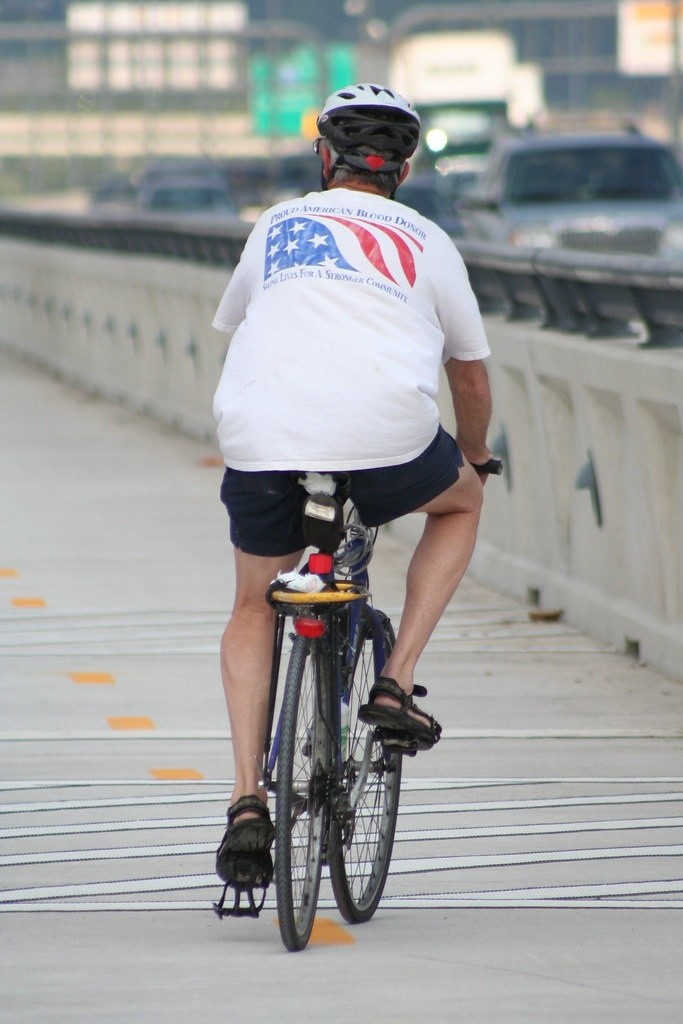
left=216, top=795, right=275, bottom=889
left=359, top=678, right=442, bottom=751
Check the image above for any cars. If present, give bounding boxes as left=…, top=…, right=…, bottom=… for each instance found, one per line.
left=458, top=116, right=683, bottom=261
left=87, top=147, right=471, bottom=238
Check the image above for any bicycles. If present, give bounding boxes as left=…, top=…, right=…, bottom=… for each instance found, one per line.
left=208, top=455, right=512, bottom=954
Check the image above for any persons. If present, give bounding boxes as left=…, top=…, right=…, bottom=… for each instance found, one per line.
left=212, top=82, right=492, bottom=887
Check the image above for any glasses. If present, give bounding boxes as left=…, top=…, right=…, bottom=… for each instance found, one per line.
left=313, top=136, right=329, bottom=154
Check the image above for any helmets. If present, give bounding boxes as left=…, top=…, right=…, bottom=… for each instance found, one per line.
left=318, top=83, right=420, bottom=172
